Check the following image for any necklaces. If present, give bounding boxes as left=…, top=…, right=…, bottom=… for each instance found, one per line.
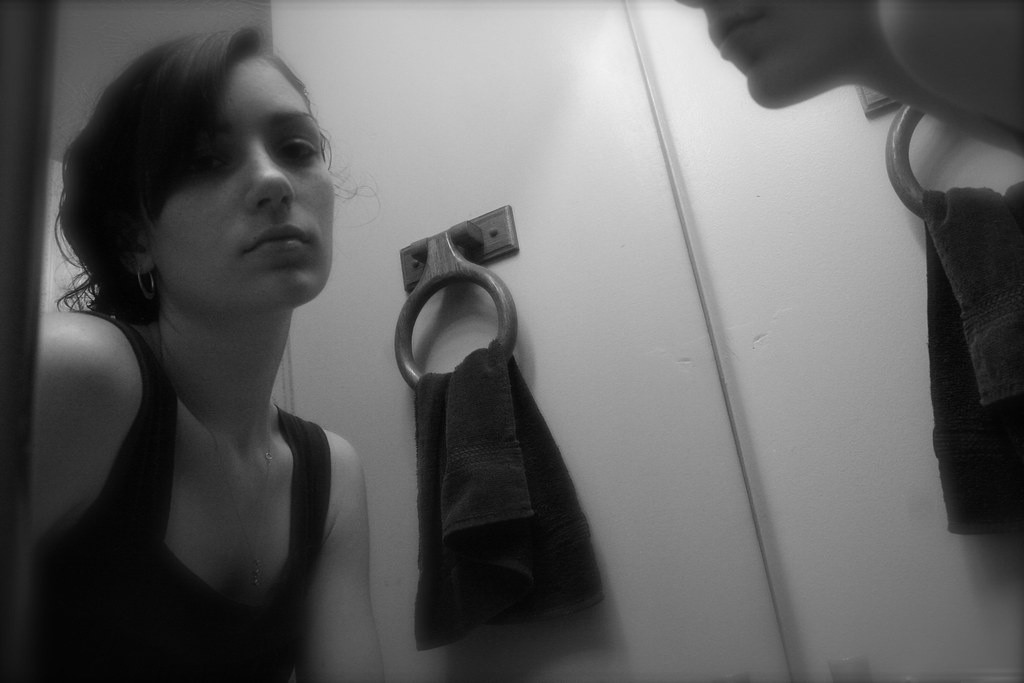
left=147, top=324, right=271, bottom=587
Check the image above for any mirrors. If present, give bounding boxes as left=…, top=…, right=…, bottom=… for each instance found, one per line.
left=15, top=0, right=807, bottom=683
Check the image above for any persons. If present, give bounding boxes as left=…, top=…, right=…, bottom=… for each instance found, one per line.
left=678, top=0, right=1024, bottom=161
left=0, top=23, right=387, bottom=683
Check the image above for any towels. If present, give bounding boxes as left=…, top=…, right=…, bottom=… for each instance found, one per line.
left=907, top=183, right=1024, bottom=539
left=412, top=338, right=605, bottom=654
